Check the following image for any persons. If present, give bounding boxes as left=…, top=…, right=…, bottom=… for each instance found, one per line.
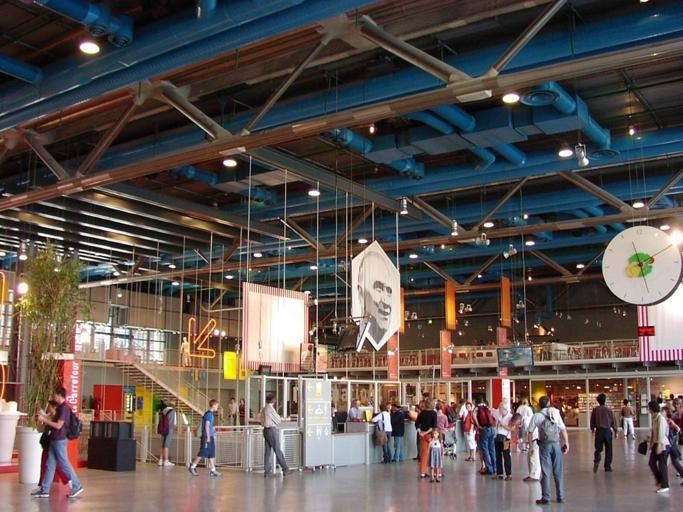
left=31, top=386, right=84, bottom=499
left=331, top=403, right=336, bottom=419
left=349, top=398, right=363, bottom=422
left=414, top=396, right=458, bottom=483
left=619, top=398, right=637, bottom=440
left=227, top=397, right=237, bottom=421
left=238, top=398, right=246, bottom=424
left=358, top=253, right=394, bottom=340
left=645, top=394, right=682, bottom=493
left=261, top=393, right=298, bottom=478
left=180, top=336, right=190, bottom=365
left=157, top=400, right=175, bottom=467
left=371, top=402, right=405, bottom=464
left=458, top=396, right=569, bottom=505
left=30, top=401, right=75, bottom=495
left=187, top=399, right=220, bottom=476
left=590, top=393, right=618, bottom=472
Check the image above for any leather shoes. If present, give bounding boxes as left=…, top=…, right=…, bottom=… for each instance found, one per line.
left=523, top=477, right=537, bottom=481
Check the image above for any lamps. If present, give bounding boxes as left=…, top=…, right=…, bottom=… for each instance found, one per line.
left=475, top=232, right=491, bottom=245
left=450, top=191, right=459, bottom=236
left=573, top=130, right=591, bottom=168
left=503, top=244, right=518, bottom=259
left=557, top=142, right=573, bottom=158
left=399, top=197, right=410, bottom=215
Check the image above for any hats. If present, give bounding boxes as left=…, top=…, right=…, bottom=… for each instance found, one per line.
left=637, top=442, right=647, bottom=455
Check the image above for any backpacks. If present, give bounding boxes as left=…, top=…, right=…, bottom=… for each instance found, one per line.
left=63, top=403, right=82, bottom=440
left=475, top=405, right=495, bottom=427
left=157, top=409, right=172, bottom=436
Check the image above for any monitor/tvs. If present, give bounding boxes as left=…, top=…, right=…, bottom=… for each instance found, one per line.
left=496, top=347, right=534, bottom=368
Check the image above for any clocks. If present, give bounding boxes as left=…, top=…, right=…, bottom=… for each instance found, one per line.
left=601, top=224, right=683, bottom=307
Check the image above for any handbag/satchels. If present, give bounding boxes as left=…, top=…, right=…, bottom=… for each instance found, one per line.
left=373, top=431, right=387, bottom=446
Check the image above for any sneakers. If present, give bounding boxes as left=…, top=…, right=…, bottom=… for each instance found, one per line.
left=188, top=464, right=198, bottom=476
left=535, top=499, right=550, bottom=505
left=66, top=487, right=84, bottom=498
left=210, top=471, right=221, bottom=476
left=158, top=460, right=163, bottom=466
left=30, top=491, right=49, bottom=498
left=283, top=469, right=296, bottom=476
left=164, top=460, right=175, bottom=467
left=263, top=472, right=281, bottom=478
left=655, top=487, right=669, bottom=493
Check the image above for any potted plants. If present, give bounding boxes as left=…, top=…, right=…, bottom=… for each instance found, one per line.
left=8, top=237, right=93, bottom=489
